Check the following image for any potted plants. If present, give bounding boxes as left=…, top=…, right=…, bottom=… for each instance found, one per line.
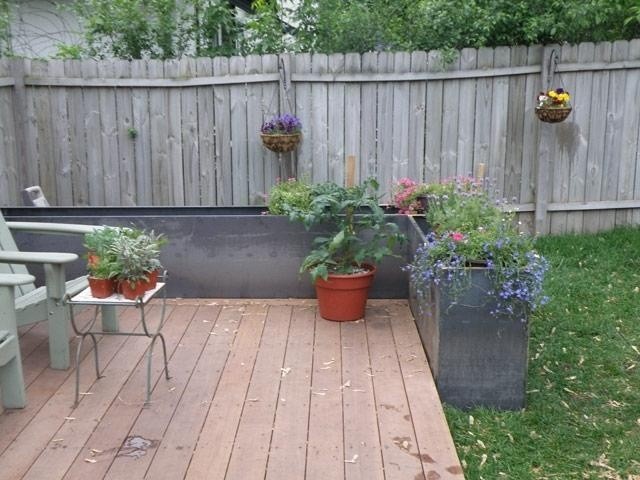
left=299, top=178, right=411, bottom=323
left=78, top=225, right=168, bottom=300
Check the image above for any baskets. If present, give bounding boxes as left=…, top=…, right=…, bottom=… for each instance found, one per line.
left=535, top=108, right=571, bottom=123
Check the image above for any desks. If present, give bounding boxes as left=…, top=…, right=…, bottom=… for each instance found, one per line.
left=57, top=276, right=176, bottom=411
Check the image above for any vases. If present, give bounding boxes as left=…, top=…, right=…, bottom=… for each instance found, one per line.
left=534, top=107, right=573, bottom=124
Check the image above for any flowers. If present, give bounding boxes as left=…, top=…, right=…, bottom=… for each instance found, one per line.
left=394, top=175, right=551, bottom=323
left=256, top=176, right=310, bottom=221
left=537, top=87, right=570, bottom=108
left=261, top=111, right=301, bottom=132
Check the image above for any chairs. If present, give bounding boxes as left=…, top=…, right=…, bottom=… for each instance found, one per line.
left=20, top=184, right=50, bottom=208
left=0, top=210, right=139, bottom=374
left=0, top=270, right=40, bottom=417
